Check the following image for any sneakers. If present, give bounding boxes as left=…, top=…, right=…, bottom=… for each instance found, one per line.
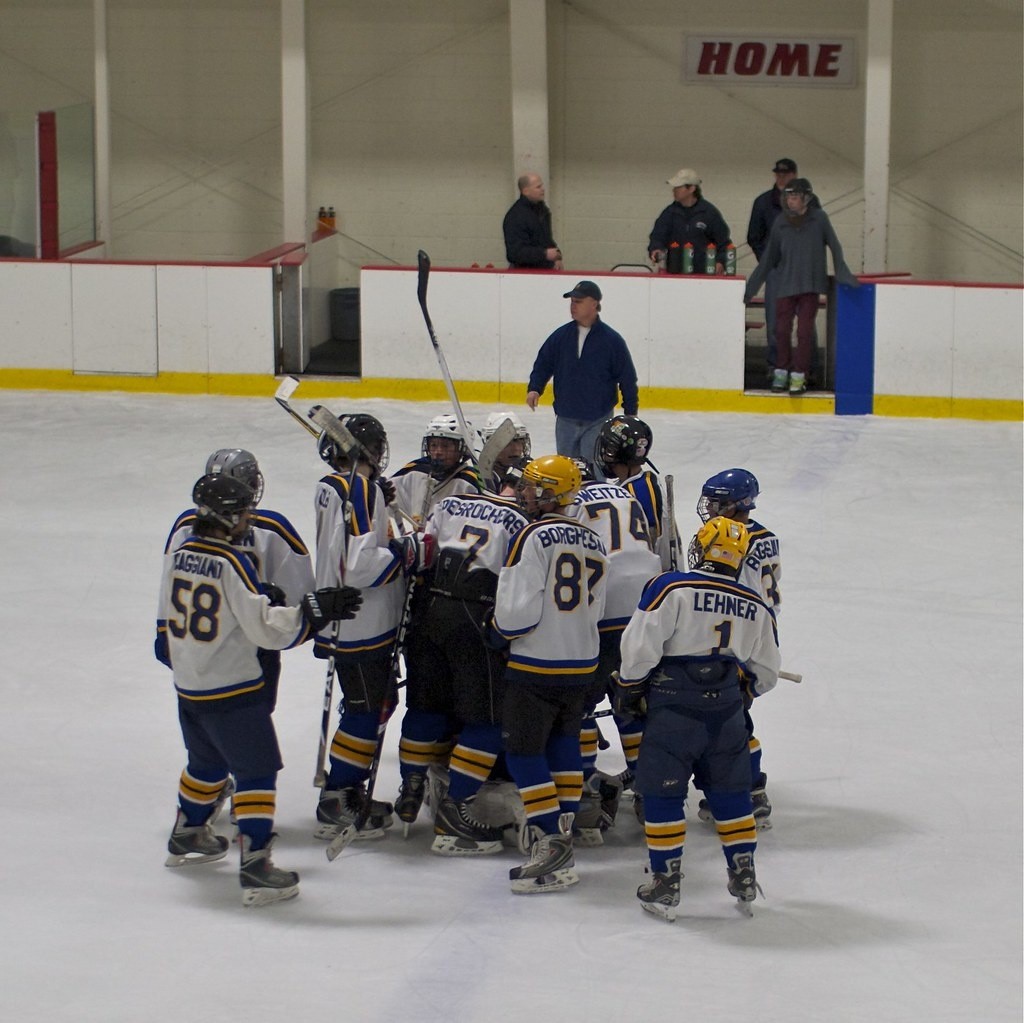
left=772, top=368, right=788, bottom=392
left=789, top=372, right=807, bottom=395
left=165, top=806, right=229, bottom=867
left=311, top=733, right=772, bottom=922
left=238, top=832, right=300, bottom=906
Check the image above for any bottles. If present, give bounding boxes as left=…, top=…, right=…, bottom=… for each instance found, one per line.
left=705, top=243, right=716, bottom=275
left=725, top=243, right=736, bottom=276
left=326, top=207, right=335, bottom=230
left=319, top=206, right=326, bottom=230
left=668, top=242, right=680, bottom=273
left=681, top=242, right=695, bottom=274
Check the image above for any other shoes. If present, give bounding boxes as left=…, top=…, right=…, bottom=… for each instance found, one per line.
left=229, top=791, right=240, bottom=848
left=207, top=778, right=235, bottom=824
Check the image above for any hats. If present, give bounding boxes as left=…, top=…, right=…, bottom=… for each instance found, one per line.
left=772, top=159, right=797, bottom=173
left=563, top=281, right=602, bottom=301
left=784, top=177, right=812, bottom=196
left=666, top=169, right=702, bottom=187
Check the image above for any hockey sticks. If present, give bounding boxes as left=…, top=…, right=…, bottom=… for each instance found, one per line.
left=414, top=249, right=479, bottom=468
left=614, top=767, right=634, bottom=792
left=324, top=564, right=420, bottom=862
left=479, top=418, right=611, bottom=751
left=273, top=375, right=420, bottom=527
left=666, top=475, right=680, bottom=576
left=780, top=670, right=804, bottom=684
left=581, top=704, right=642, bottom=721
left=308, top=403, right=362, bottom=788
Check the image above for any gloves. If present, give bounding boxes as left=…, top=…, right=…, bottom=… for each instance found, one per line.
left=301, top=585, right=364, bottom=633
left=740, top=679, right=754, bottom=711
left=481, top=606, right=511, bottom=654
left=389, top=532, right=440, bottom=580
left=260, top=583, right=287, bottom=607
left=615, top=680, right=650, bottom=723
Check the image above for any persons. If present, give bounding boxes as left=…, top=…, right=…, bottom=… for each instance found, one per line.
left=743, top=178, right=858, bottom=395
left=314, top=413, right=782, bottom=893
left=747, top=158, right=822, bottom=379
left=503, top=172, right=564, bottom=271
left=155, top=446, right=364, bottom=906
left=526, top=281, right=639, bottom=466
left=613, top=516, right=781, bottom=921
left=648, top=167, right=731, bottom=276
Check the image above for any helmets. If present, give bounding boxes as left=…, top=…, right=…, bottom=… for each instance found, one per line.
left=688, top=516, right=749, bottom=577
left=594, top=415, right=659, bottom=480
left=206, top=449, right=265, bottom=510
left=697, top=468, right=761, bottom=526
left=332, top=414, right=390, bottom=481
left=515, top=455, right=582, bottom=516
left=477, top=411, right=531, bottom=469
left=193, top=473, right=258, bottom=540
left=421, top=414, right=476, bottom=472
left=571, top=456, right=595, bottom=480
